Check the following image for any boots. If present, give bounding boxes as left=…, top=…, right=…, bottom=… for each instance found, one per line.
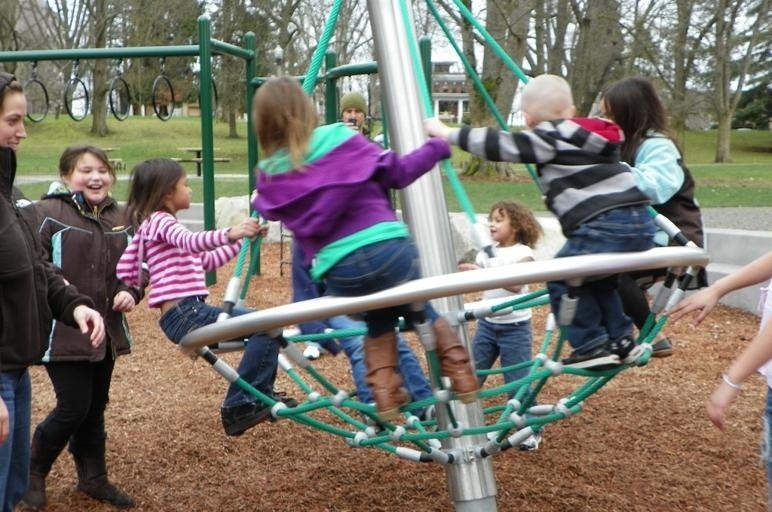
left=364, top=331, right=411, bottom=422
left=69, top=432, right=133, bottom=505
left=24, top=425, right=63, bottom=508
left=432, top=318, right=478, bottom=404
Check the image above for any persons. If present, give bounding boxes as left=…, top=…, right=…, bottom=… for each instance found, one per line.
left=460, top=198, right=549, bottom=455
left=248, top=73, right=482, bottom=424
left=336, top=89, right=375, bottom=139
left=2, top=72, right=109, bottom=511
left=666, top=248, right=772, bottom=511
left=586, top=73, right=704, bottom=359
left=111, top=156, right=262, bottom=439
left=418, top=72, right=667, bottom=372
left=22, top=144, right=148, bottom=508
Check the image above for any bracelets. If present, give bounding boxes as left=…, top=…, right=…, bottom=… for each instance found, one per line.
left=721, top=372, right=744, bottom=394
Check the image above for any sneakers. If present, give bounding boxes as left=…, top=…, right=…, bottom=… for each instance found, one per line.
left=618, top=336, right=644, bottom=364
left=303, top=345, right=320, bottom=358
left=520, top=433, right=542, bottom=450
left=221, top=395, right=297, bottom=436
left=652, top=340, right=675, bottom=357
left=561, top=345, right=621, bottom=368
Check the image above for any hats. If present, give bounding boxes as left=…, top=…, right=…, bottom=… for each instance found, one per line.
left=340, top=91, right=367, bottom=118
